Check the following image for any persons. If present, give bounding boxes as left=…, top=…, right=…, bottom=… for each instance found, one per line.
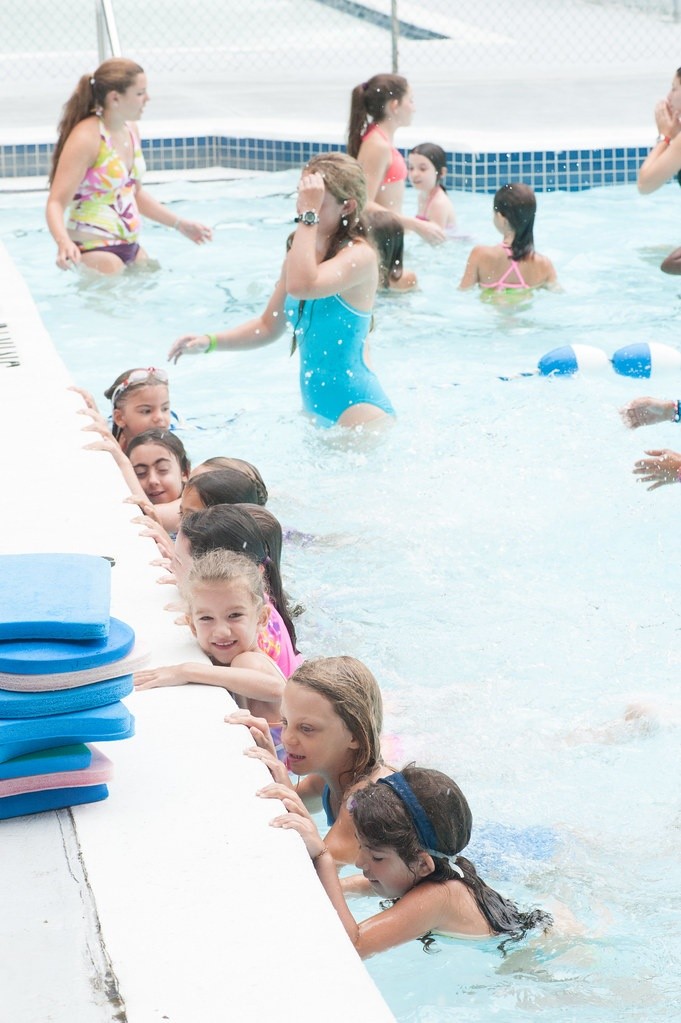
left=349, top=73, right=445, bottom=289
left=168, top=151, right=395, bottom=427
left=462, top=181, right=556, bottom=290
left=164, top=503, right=303, bottom=674
left=257, top=765, right=496, bottom=947
left=661, top=246, right=681, bottom=274
left=408, top=143, right=455, bottom=231
left=140, top=471, right=258, bottom=557
left=619, top=396, right=681, bottom=491
left=68, top=368, right=170, bottom=453
left=124, top=456, right=268, bottom=541
left=638, top=66, right=681, bottom=195
left=131, top=549, right=288, bottom=764
left=45, top=58, right=212, bottom=273
left=150, top=503, right=281, bottom=585
left=225, top=656, right=396, bottom=861
left=82, top=423, right=191, bottom=504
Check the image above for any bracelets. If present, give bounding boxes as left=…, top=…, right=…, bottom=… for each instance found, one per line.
left=205, top=333, right=218, bottom=354
left=311, top=847, right=328, bottom=862
left=175, top=217, right=182, bottom=229
left=675, top=400, right=681, bottom=423
left=672, top=400, right=679, bottom=422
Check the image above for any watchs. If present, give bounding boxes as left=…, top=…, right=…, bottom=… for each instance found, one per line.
left=294, top=209, right=319, bottom=225
left=656, top=134, right=671, bottom=144
